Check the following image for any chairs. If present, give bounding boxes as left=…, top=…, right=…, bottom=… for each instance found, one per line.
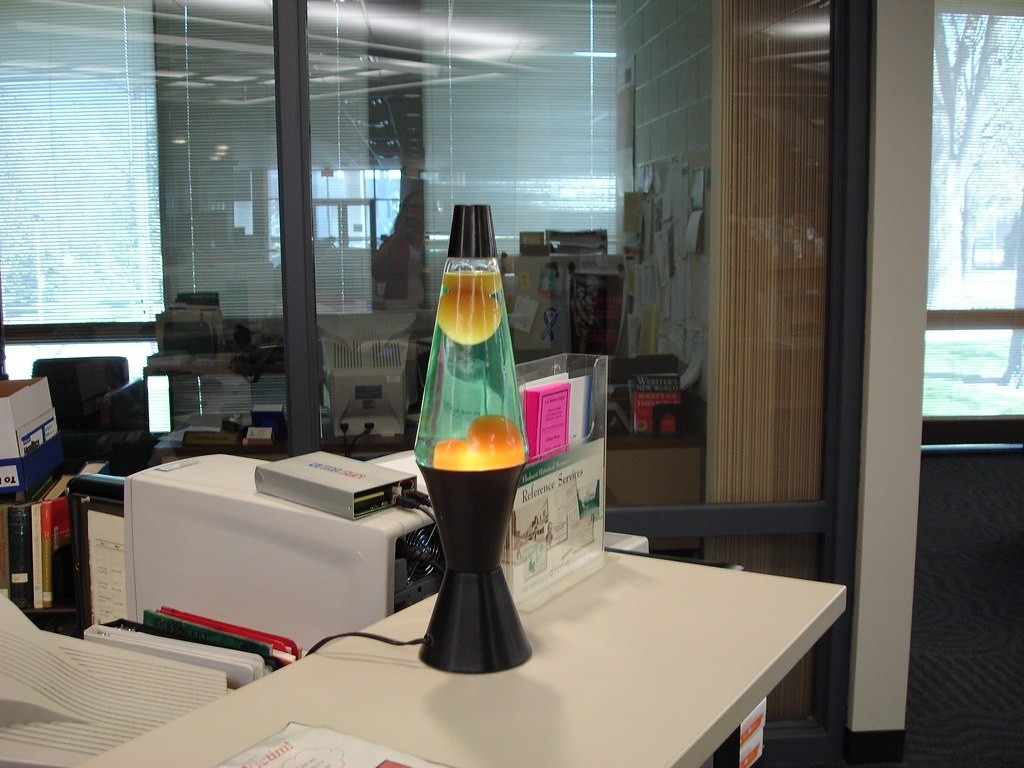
left=31, top=357, right=146, bottom=475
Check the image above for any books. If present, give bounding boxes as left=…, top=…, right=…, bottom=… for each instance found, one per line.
left=518, top=372, right=593, bottom=464
left=156, top=291, right=224, bottom=356
left=0, top=461, right=302, bottom=768
left=627, top=372, right=682, bottom=437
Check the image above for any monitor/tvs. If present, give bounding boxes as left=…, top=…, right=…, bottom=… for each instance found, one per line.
left=65, top=474, right=127, bottom=639
left=315, top=313, right=419, bottom=436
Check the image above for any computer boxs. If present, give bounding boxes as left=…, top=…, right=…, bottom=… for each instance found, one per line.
left=122, top=453, right=447, bottom=657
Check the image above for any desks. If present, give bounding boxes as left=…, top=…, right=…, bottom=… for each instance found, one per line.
left=20, top=598, right=78, bottom=615
left=77, top=549, right=845, bottom=768
left=152, top=422, right=417, bottom=461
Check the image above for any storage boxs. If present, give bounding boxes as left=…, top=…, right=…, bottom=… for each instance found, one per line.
left=620, top=353, right=682, bottom=438
left=0, top=376, right=64, bottom=493
